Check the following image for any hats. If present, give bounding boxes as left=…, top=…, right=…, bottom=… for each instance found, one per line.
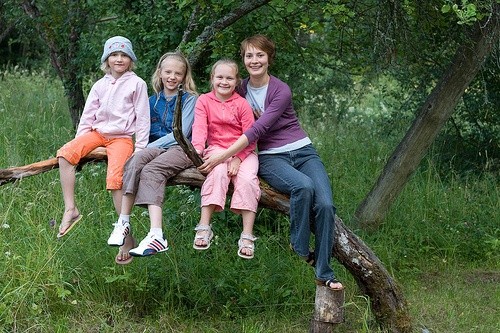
left=101, top=35, right=137, bottom=62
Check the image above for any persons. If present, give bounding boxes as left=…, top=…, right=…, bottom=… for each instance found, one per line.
left=108, top=52, right=199, bottom=256
left=197, top=35, right=344, bottom=291
left=191, top=59, right=261, bottom=259
left=57, top=36, right=150, bottom=264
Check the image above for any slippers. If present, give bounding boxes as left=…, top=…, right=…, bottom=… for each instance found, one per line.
left=57, top=208, right=83, bottom=237
left=115, top=236, right=135, bottom=263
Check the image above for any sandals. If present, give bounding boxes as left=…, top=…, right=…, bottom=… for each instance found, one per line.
left=193, top=222, right=213, bottom=249
left=238, top=231, right=257, bottom=258
left=325, top=277, right=342, bottom=290
left=290, top=242, right=317, bottom=268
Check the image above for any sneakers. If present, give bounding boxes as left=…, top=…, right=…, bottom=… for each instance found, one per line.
left=107, top=218, right=131, bottom=245
left=129, top=233, right=169, bottom=256
